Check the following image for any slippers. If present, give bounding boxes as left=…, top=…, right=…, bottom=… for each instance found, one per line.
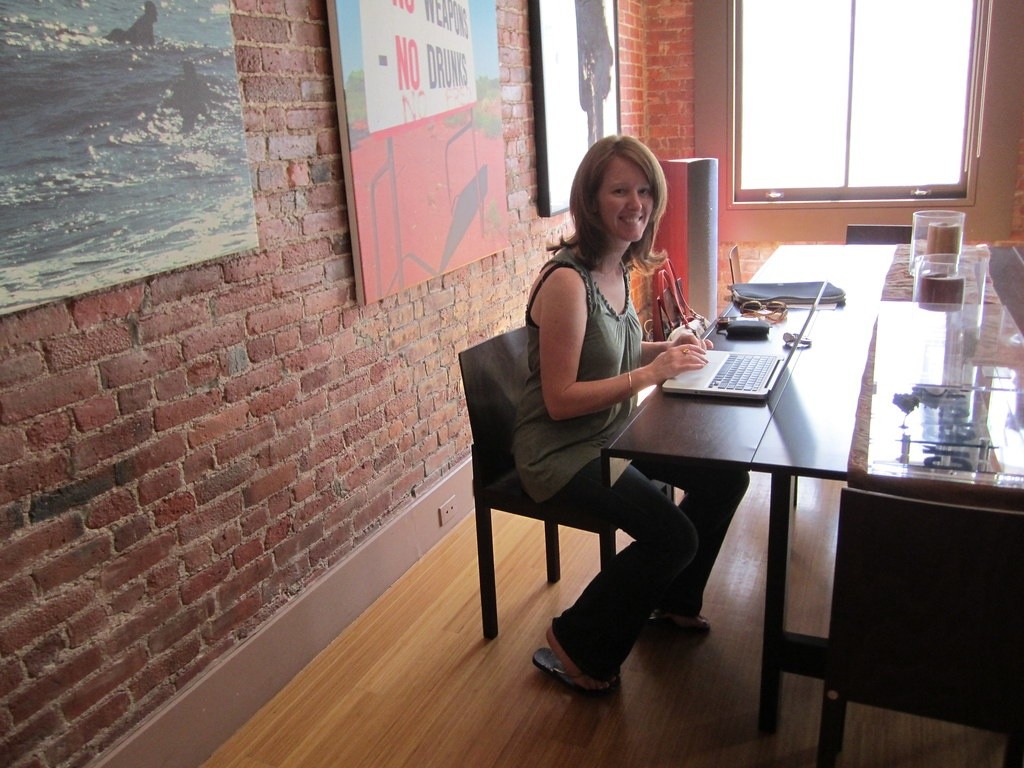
left=532, top=647, right=615, bottom=696
left=649, top=611, right=710, bottom=632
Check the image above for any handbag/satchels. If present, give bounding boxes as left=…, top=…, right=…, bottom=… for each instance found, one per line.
left=658, top=258, right=711, bottom=344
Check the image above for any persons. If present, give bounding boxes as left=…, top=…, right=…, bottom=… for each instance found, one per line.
left=514, top=132, right=715, bottom=699
left=572, top=0, right=616, bottom=148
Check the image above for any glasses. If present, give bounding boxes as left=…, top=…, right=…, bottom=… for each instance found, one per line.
left=740, top=300, right=787, bottom=321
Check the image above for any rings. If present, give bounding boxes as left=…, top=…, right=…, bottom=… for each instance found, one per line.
left=682, top=348, right=691, bottom=354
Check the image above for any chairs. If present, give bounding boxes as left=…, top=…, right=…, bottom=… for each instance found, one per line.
left=657, top=278, right=686, bottom=341
left=816, top=487, right=1024, bottom=768
left=728, top=246, right=743, bottom=286
left=845, top=224, right=927, bottom=245
left=458, top=326, right=676, bottom=643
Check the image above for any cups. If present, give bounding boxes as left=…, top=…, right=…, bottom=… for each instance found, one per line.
left=914, top=252, right=985, bottom=303
left=909, top=210, right=965, bottom=277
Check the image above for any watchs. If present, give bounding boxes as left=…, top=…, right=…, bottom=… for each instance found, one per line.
left=782, top=332, right=812, bottom=349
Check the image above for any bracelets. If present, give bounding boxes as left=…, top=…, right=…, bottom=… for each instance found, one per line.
left=627, top=370, right=636, bottom=401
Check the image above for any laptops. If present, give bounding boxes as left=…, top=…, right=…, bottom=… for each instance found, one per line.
left=662, top=279, right=829, bottom=400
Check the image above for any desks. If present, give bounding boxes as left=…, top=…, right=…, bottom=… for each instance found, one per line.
left=601, top=245, right=1024, bottom=730
